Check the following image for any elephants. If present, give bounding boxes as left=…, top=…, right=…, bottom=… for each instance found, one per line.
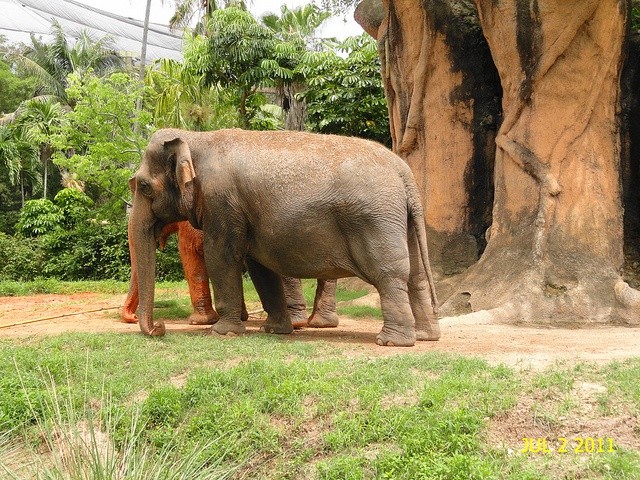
left=119, top=127, right=442, bottom=348
